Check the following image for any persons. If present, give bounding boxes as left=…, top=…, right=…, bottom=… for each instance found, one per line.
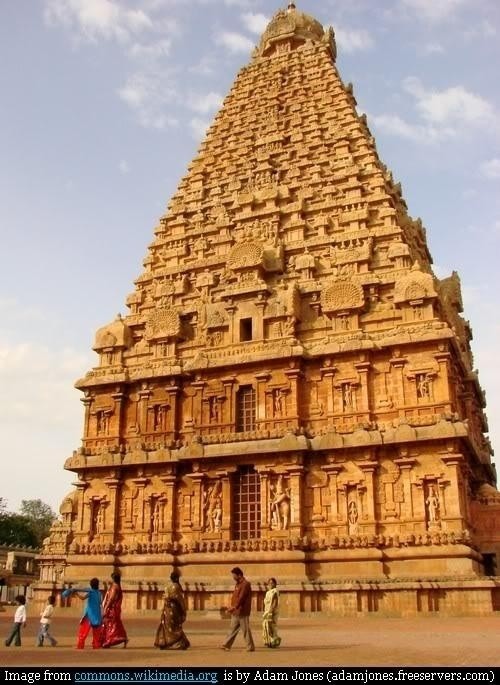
left=416, top=375, right=427, bottom=397
left=426, top=486, right=438, bottom=521
left=271, top=477, right=289, bottom=530
left=36, top=595, right=59, bottom=647
left=222, top=567, right=254, bottom=652
left=152, top=505, right=159, bottom=533
left=156, top=408, right=162, bottom=424
left=5, top=595, right=27, bottom=648
left=204, top=487, right=222, bottom=533
left=211, top=398, right=216, bottom=418
left=94, top=510, right=101, bottom=533
left=274, top=390, right=281, bottom=411
left=72, top=577, right=105, bottom=649
left=349, top=503, right=357, bottom=523
left=100, top=572, right=128, bottom=649
left=344, top=385, right=350, bottom=406
left=98, top=412, right=106, bottom=432
left=153, top=571, right=191, bottom=651
left=106, top=48, right=422, bottom=366
left=262, top=578, right=281, bottom=647
